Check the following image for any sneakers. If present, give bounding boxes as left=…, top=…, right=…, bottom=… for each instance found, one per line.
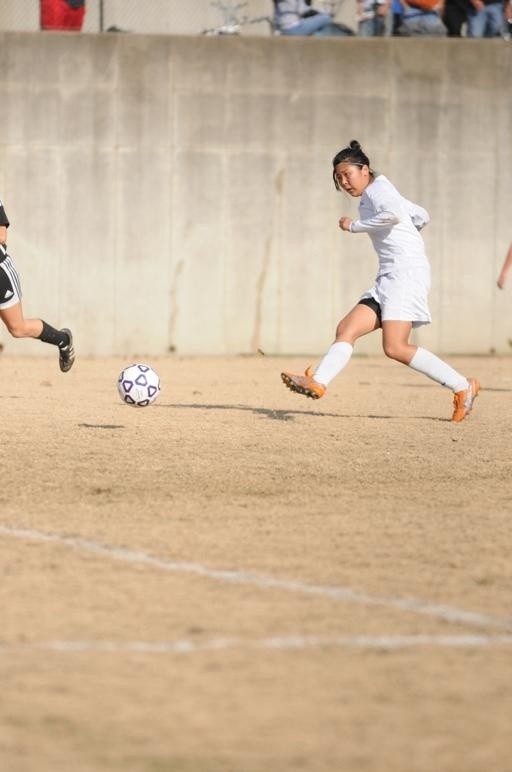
left=452, top=377, right=480, bottom=422
left=280, top=365, right=327, bottom=400
left=59, top=328, right=75, bottom=373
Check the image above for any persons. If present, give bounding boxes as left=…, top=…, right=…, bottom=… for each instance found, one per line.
left=273, top=1, right=512, bottom=44
left=497, top=246, right=511, bottom=289
left=0, top=200, right=77, bottom=374
left=279, top=139, right=483, bottom=425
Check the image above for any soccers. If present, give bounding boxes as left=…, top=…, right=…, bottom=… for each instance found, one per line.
left=118, top=363, right=161, bottom=406
